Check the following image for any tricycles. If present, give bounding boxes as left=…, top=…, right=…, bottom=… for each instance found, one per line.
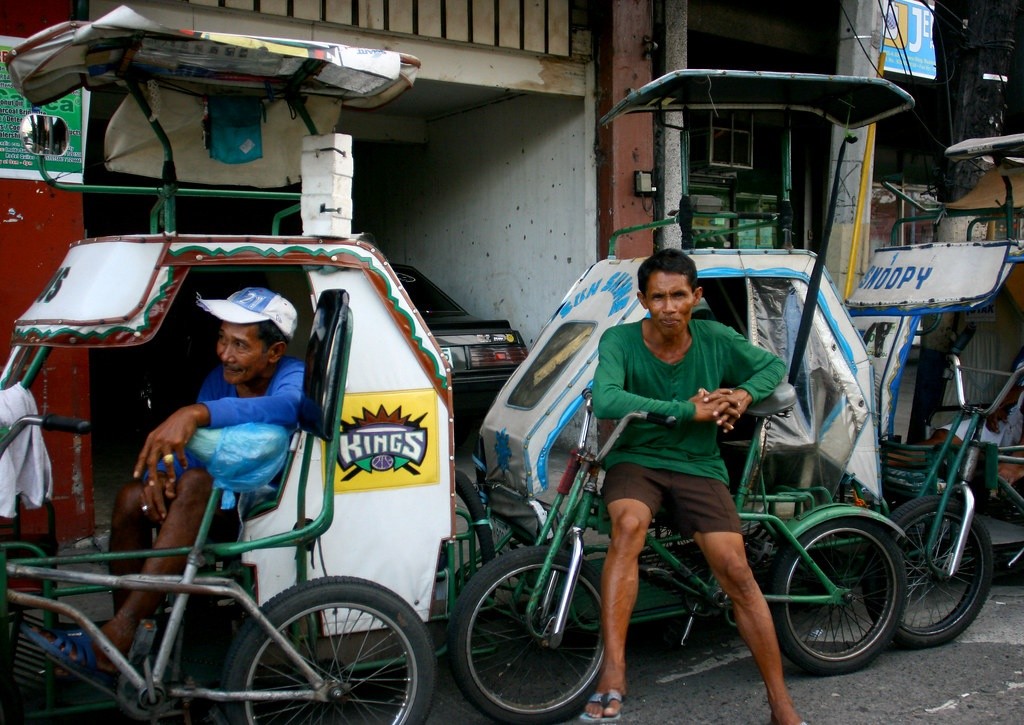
left=0, top=10, right=459, bottom=725
left=447, top=67, right=918, bottom=725
left=859, top=134, right=1024, bottom=646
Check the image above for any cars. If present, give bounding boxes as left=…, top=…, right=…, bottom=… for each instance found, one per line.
left=368, top=260, right=530, bottom=442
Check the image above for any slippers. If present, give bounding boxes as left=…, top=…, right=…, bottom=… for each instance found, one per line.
left=21, top=626, right=119, bottom=699
left=579, top=691, right=622, bottom=723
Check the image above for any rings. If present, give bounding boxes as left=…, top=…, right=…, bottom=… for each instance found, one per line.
left=163, top=454, right=174, bottom=464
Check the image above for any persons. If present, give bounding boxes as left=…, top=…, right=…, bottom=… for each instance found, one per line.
left=879, top=375, right=1024, bottom=465
left=31, top=287, right=308, bottom=676
left=582, top=249, right=807, bottom=725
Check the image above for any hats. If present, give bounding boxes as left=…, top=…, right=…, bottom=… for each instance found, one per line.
left=196, top=287, right=298, bottom=341
